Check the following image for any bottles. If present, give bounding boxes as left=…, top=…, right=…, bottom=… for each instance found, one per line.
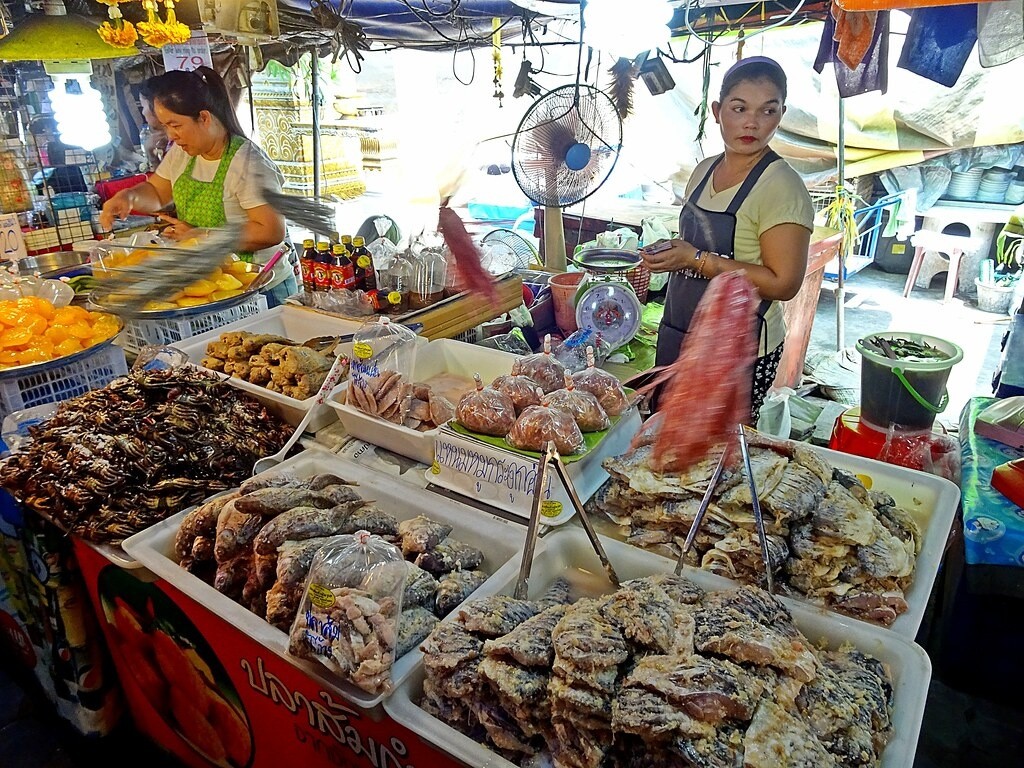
left=139, top=123, right=154, bottom=156
left=1003, top=275, right=1014, bottom=287
left=300, top=232, right=401, bottom=312
left=995, top=278, right=1006, bottom=287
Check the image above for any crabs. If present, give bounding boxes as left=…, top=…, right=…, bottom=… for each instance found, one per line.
left=0, top=366, right=302, bottom=550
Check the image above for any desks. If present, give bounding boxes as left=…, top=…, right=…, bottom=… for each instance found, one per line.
left=532, top=200, right=845, bottom=391
left=949, top=395, right=1024, bottom=652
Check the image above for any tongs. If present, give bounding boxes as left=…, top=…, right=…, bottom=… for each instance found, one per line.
left=514, top=439, right=619, bottom=600
left=674, top=424, right=774, bottom=593
left=302, top=323, right=423, bottom=385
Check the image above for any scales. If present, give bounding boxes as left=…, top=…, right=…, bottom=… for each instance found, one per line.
left=572, top=247, right=645, bottom=360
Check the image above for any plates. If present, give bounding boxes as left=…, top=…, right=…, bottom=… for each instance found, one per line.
left=949, top=166, right=1009, bottom=203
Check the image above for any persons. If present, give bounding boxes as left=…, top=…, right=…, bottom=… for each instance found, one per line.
left=639, top=56, right=814, bottom=430
left=99, top=66, right=304, bottom=309
left=139, top=75, right=174, bottom=157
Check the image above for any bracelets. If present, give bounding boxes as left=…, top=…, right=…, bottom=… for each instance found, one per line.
left=698, top=251, right=709, bottom=274
left=694, top=249, right=703, bottom=260
left=205, top=229, right=211, bottom=238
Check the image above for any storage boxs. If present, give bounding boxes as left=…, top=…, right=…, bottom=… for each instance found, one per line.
left=0, top=209, right=961, bottom=768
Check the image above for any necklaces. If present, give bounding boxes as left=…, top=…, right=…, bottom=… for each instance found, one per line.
left=710, top=157, right=756, bottom=183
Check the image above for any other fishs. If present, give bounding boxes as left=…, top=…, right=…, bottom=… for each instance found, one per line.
left=171, top=426, right=923, bottom=767
left=199, top=328, right=349, bottom=400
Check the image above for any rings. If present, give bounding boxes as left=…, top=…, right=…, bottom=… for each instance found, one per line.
left=172, top=226, right=176, bottom=233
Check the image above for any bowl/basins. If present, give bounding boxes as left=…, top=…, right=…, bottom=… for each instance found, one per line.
left=974, top=275, right=1014, bottom=313
left=0, top=251, right=89, bottom=276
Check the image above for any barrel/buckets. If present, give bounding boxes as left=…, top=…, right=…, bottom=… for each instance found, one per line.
left=549, top=271, right=585, bottom=331
left=855, top=330, right=963, bottom=437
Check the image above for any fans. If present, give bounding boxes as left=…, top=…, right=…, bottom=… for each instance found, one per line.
left=481, top=228, right=544, bottom=269
left=510, top=84, right=623, bottom=272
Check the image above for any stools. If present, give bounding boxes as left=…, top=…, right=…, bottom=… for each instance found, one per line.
left=902, top=230, right=982, bottom=300
